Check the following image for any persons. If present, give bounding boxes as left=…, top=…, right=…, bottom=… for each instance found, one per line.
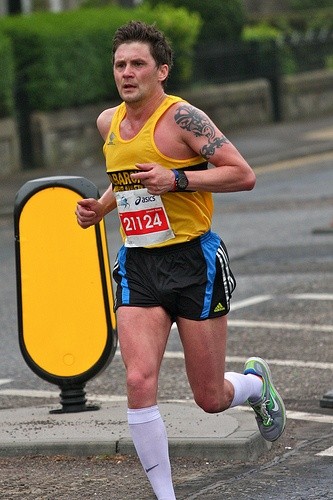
left=75, top=19, right=287, bottom=499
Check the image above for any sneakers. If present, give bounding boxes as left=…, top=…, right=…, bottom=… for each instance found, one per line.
left=240, top=356, right=287, bottom=443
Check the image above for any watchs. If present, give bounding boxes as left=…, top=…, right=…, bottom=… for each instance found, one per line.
left=172, top=169, right=189, bottom=191
